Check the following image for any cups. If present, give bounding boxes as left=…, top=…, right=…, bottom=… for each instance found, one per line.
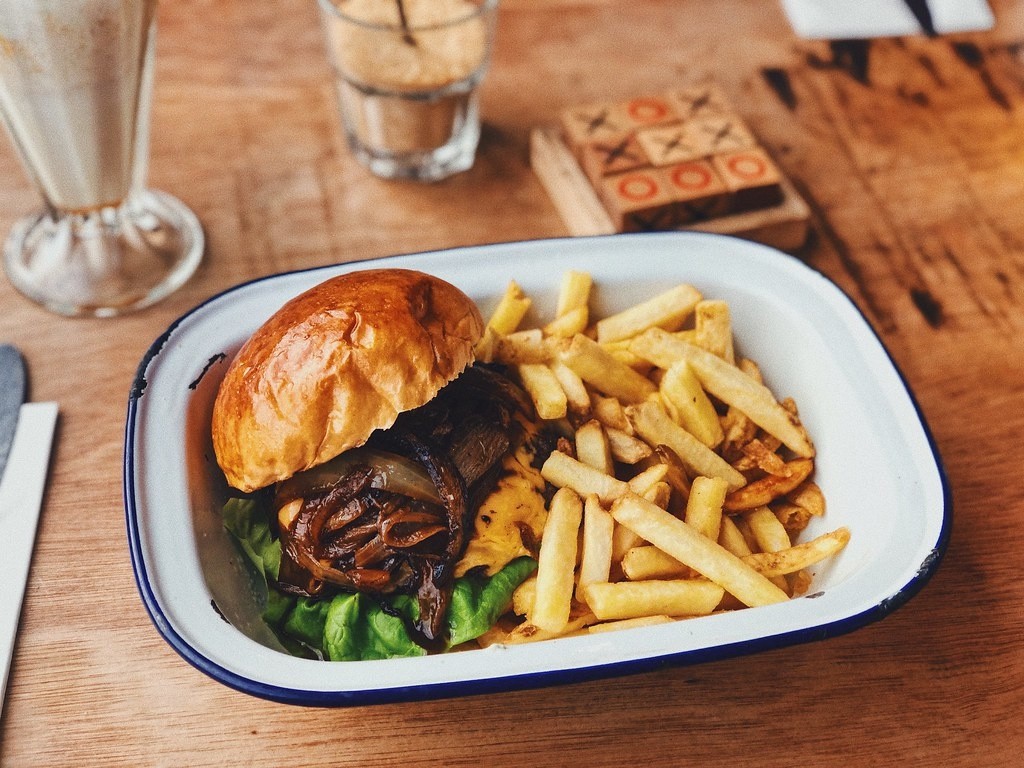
left=318, top=0, right=498, bottom=183
left=0, top=0, right=205, bottom=318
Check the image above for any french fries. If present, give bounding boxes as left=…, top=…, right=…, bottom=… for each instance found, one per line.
left=476, top=268, right=849, bottom=643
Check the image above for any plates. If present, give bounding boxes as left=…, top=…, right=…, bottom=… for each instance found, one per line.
left=120, top=230, right=954, bottom=708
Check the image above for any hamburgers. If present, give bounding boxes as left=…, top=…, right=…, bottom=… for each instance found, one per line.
left=210, top=267, right=568, bottom=661
